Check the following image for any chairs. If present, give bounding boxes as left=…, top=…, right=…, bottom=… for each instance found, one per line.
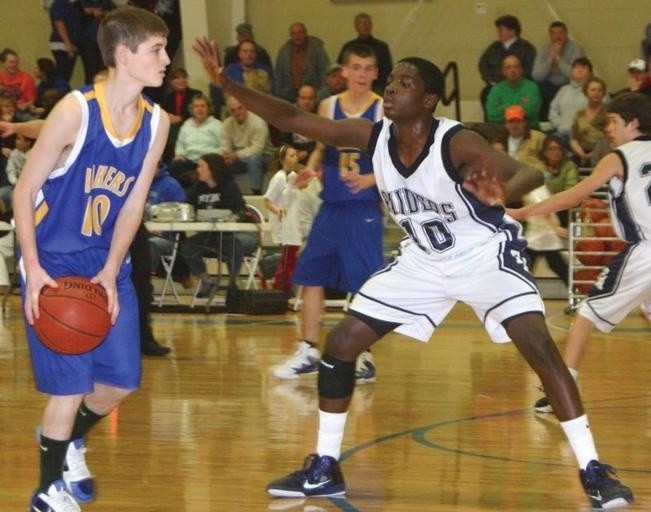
left=151, top=231, right=183, bottom=307
left=189, top=202, right=267, bottom=314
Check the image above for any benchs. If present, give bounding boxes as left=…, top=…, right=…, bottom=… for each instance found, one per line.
left=528, top=249, right=575, bottom=301
left=204, top=168, right=277, bottom=294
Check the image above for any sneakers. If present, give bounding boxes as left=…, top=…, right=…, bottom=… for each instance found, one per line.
left=535, top=396, right=553, bottom=414
left=351, top=349, right=378, bottom=386
left=578, top=460, right=635, bottom=511
left=29, top=480, right=83, bottom=511
left=273, top=341, right=322, bottom=381
left=196, top=276, right=215, bottom=302
left=266, top=456, right=346, bottom=499
left=141, top=335, right=172, bottom=361
left=61, top=437, right=96, bottom=502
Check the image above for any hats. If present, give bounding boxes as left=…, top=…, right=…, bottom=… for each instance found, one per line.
left=236, top=22, right=252, bottom=33
left=503, top=105, right=526, bottom=123
left=326, top=63, right=341, bottom=75
left=626, top=58, right=647, bottom=72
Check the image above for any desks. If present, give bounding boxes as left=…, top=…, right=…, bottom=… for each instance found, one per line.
left=1, top=221, right=275, bottom=314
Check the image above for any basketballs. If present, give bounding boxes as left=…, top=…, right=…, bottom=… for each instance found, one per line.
left=576, top=196, right=629, bottom=293
left=31, top=275, right=112, bottom=354
